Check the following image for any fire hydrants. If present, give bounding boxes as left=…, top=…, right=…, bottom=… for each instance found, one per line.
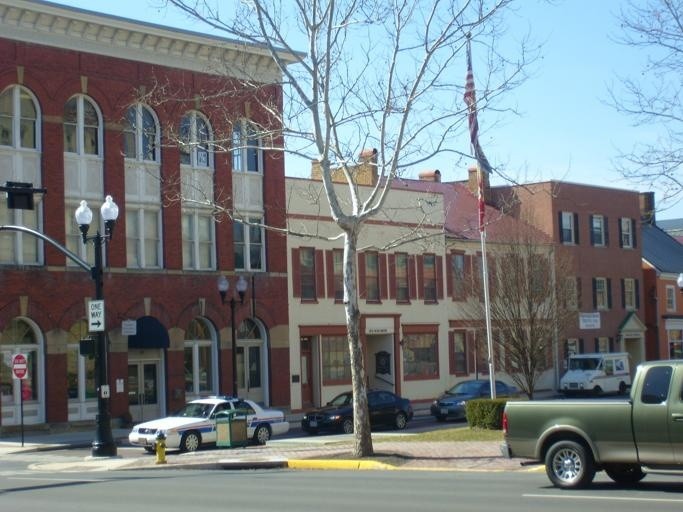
left=154, top=430, right=167, bottom=464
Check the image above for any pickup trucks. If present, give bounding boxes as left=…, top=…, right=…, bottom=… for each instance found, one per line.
left=502, top=360, right=683, bottom=489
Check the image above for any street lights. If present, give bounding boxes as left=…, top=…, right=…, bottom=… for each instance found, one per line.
left=75, top=196, right=119, bottom=457
left=217, top=270, right=248, bottom=398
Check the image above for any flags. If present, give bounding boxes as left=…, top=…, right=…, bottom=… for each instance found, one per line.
left=462, top=33, right=494, bottom=178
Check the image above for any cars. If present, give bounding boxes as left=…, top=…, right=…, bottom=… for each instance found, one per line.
left=128, top=393, right=290, bottom=452
left=430, top=380, right=517, bottom=421
left=302, top=389, right=413, bottom=433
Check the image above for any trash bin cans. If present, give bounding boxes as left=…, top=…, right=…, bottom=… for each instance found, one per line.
left=215, top=410, right=248, bottom=449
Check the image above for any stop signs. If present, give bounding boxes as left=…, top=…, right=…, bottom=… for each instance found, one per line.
left=11, top=353, right=28, bottom=380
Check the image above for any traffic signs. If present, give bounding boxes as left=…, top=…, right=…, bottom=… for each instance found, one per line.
left=89, top=300, right=105, bottom=332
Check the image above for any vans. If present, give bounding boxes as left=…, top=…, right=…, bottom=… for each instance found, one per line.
left=557, top=352, right=632, bottom=397
